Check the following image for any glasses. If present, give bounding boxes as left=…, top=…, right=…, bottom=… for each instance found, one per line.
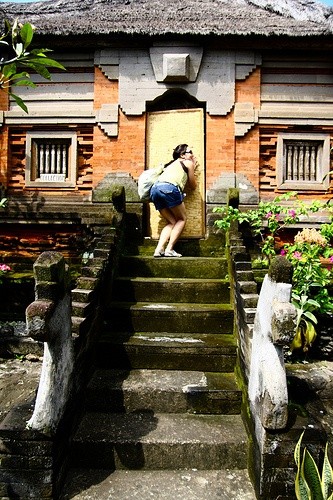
left=182, top=150, right=193, bottom=155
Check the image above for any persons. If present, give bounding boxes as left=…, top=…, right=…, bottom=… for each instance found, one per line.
left=150, top=143, right=199, bottom=257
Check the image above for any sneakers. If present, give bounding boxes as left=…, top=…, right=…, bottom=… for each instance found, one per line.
left=153, top=246, right=165, bottom=257
left=164, top=249, right=183, bottom=257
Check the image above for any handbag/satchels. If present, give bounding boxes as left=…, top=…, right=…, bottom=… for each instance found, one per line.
left=137, top=157, right=177, bottom=203
